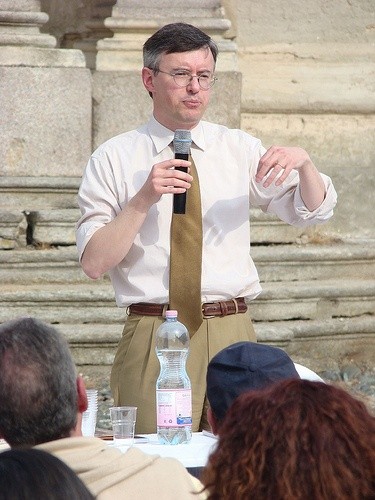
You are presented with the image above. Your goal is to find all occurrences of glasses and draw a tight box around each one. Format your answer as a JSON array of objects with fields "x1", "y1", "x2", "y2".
[{"x1": 151, "y1": 68, "x2": 218, "y2": 89}]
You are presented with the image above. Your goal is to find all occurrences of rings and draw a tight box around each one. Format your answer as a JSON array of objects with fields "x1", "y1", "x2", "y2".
[{"x1": 276, "y1": 163, "x2": 284, "y2": 168}]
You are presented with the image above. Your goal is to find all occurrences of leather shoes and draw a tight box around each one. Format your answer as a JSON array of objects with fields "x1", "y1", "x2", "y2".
[{"x1": 129, "y1": 297, "x2": 248, "y2": 317}]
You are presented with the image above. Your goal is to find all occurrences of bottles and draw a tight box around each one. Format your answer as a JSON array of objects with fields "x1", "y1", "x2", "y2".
[{"x1": 156, "y1": 310, "x2": 192, "y2": 446}]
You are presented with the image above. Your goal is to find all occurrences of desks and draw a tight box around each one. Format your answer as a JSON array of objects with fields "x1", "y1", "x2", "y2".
[{"x1": 102, "y1": 430, "x2": 218, "y2": 468}]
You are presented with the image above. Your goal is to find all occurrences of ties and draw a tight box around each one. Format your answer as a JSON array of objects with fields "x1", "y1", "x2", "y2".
[{"x1": 168, "y1": 146, "x2": 204, "y2": 346}]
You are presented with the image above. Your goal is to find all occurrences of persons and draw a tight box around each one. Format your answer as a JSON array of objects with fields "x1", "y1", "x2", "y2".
[
  {"x1": 0, "y1": 318, "x2": 374, "y2": 500},
  {"x1": 76, "y1": 23, "x2": 338, "y2": 434}
]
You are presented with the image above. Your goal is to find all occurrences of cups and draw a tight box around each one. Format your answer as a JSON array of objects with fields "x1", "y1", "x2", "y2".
[
  {"x1": 108, "y1": 406, "x2": 138, "y2": 444},
  {"x1": 81, "y1": 390, "x2": 98, "y2": 437}
]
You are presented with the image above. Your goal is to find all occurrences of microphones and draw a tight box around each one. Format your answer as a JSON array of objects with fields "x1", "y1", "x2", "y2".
[{"x1": 172, "y1": 129, "x2": 191, "y2": 214}]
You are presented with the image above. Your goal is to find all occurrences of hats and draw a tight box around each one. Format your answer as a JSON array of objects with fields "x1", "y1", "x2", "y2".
[{"x1": 206, "y1": 341, "x2": 301, "y2": 427}]
